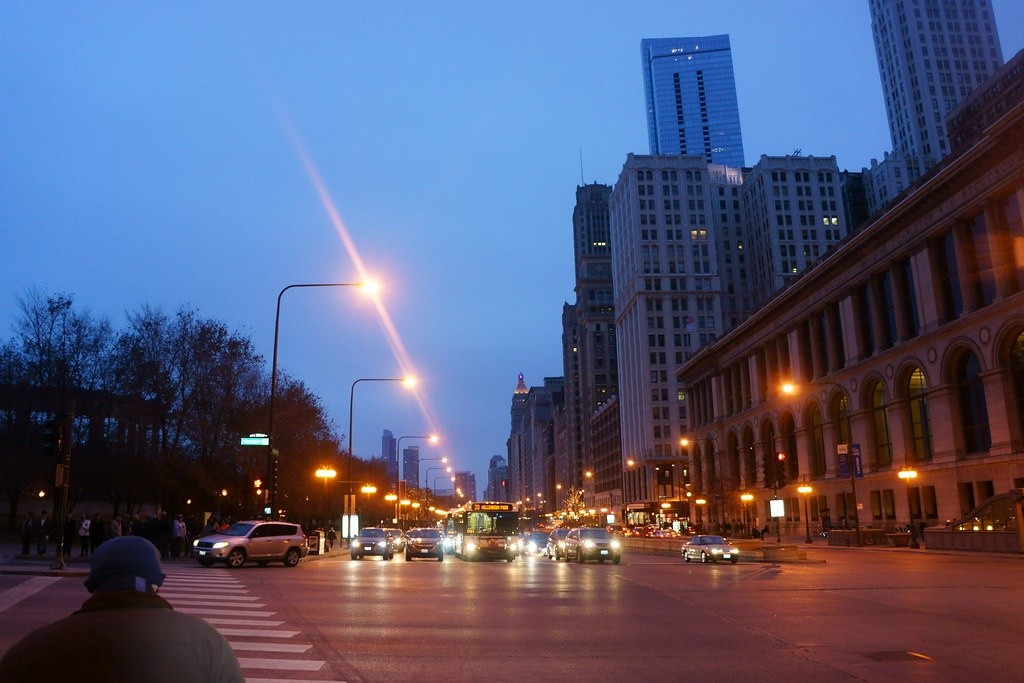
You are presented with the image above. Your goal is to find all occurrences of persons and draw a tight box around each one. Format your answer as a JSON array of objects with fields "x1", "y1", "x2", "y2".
[
  {"x1": 734, "y1": 522, "x2": 744, "y2": 534},
  {"x1": 0, "y1": 535, "x2": 245, "y2": 683},
  {"x1": 110, "y1": 513, "x2": 197, "y2": 557},
  {"x1": 204, "y1": 517, "x2": 230, "y2": 535},
  {"x1": 22, "y1": 510, "x2": 50, "y2": 558},
  {"x1": 327, "y1": 527, "x2": 336, "y2": 547},
  {"x1": 722, "y1": 522, "x2": 732, "y2": 533},
  {"x1": 78, "y1": 513, "x2": 104, "y2": 558},
  {"x1": 63, "y1": 511, "x2": 77, "y2": 557},
  {"x1": 919, "y1": 519, "x2": 927, "y2": 541}
]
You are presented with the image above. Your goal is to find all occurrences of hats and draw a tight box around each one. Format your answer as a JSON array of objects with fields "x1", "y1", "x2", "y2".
[{"x1": 84, "y1": 535, "x2": 166, "y2": 592}]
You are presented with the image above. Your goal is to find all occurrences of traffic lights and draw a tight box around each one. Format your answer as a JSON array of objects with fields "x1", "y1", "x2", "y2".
[
  {"x1": 773, "y1": 451, "x2": 786, "y2": 487},
  {"x1": 250, "y1": 476, "x2": 262, "y2": 500},
  {"x1": 762, "y1": 453, "x2": 774, "y2": 490}
]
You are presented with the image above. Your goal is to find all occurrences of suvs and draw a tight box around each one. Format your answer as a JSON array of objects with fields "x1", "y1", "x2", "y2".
[{"x1": 192, "y1": 519, "x2": 311, "y2": 568}]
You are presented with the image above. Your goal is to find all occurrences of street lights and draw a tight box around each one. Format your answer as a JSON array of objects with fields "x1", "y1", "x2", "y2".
[
  {"x1": 444, "y1": 487, "x2": 461, "y2": 508},
  {"x1": 625, "y1": 459, "x2": 668, "y2": 496},
  {"x1": 316, "y1": 470, "x2": 335, "y2": 522},
  {"x1": 417, "y1": 457, "x2": 449, "y2": 525},
  {"x1": 782, "y1": 381, "x2": 861, "y2": 546},
  {"x1": 267, "y1": 281, "x2": 383, "y2": 515},
  {"x1": 412, "y1": 503, "x2": 420, "y2": 521},
  {"x1": 677, "y1": 438, "x2": 727, "y2": 536},
  {"x1": 399, "y1": 499, "x2": 411, "y2": 527},
  {"x1": 694, "y1": 497, "x2": 706, "y2": 535},
  {"x1": 343, "y1": 375, "x2": 420, "y2": 546},
  {"x1": 584, "y1": 471, "x2": 613, "y2": 513},
  {"x1": 425, "y1": 466, "x2": 452, "y2": 526},
  {"x1": 385, "y1": 494, "x2": 396, "y2": 525},
  {"x1": 740, "y1": 494, "x2": 754, "y2": 540},
  {"x1": 432, "y1": 476, "x2": 456, "y2": 507},
  {"x1": 361, "y1": 485, "x2": 377, "y2": 524},
  {"x1": 897, "y1": 468, "x2": 920, "y2": 549},
  {"x1": 796, "y1": 473, "x2": 815, "y2": 544},
  {"x1": 394, "y1": 436, "x2": 441, "y2": 527}
]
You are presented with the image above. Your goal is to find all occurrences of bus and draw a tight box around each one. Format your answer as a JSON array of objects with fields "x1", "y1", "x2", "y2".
[{"x1": 440, "y1": 499, "x2": 521, "y2": 562}]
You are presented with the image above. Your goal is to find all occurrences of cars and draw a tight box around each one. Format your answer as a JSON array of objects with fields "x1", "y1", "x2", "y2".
[
  {"x1": 680, "y1": 534, "x2": 740, "y2": 565},
  {"x1": 518, "y1": 524, "x2": 681, "y2": 565},
  {"x1": 349, "y1": 526, "x2": 446, "y2": 563}
]
